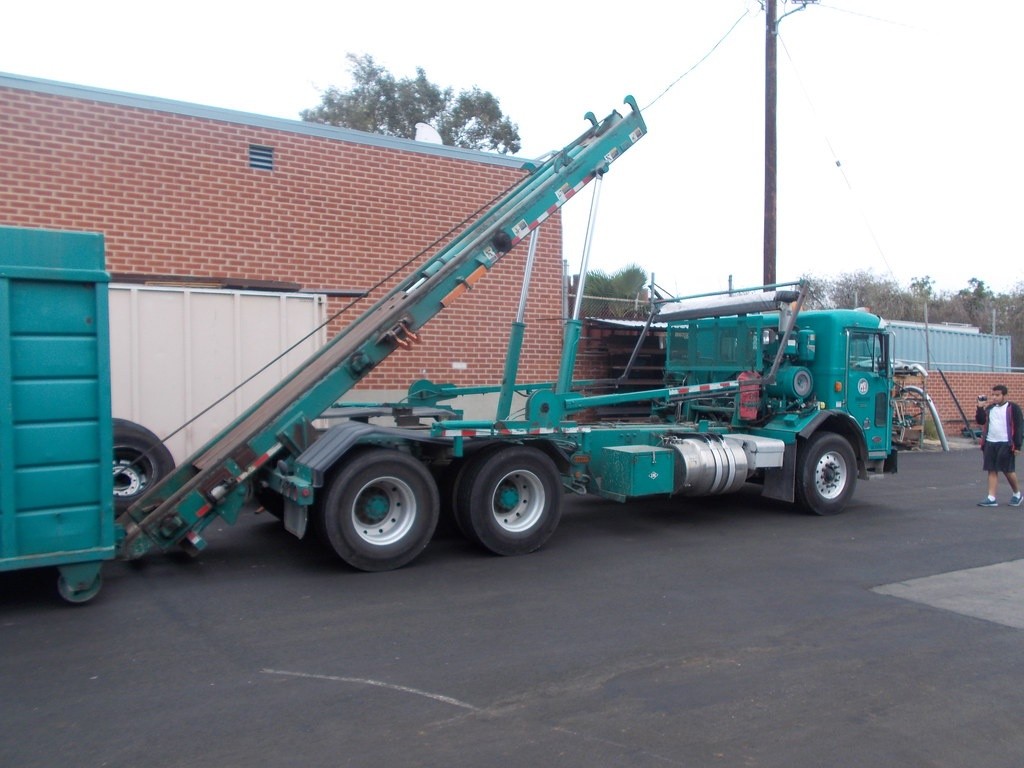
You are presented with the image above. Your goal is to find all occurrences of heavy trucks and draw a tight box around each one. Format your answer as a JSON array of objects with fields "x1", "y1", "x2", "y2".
[{"x1": 116, "y1": 94, "x2": 899, "y2": 573}]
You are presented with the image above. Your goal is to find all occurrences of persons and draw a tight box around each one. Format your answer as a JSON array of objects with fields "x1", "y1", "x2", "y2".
[{"x1": 976, "y1": 386, "x2": 1024, "y2": 507}]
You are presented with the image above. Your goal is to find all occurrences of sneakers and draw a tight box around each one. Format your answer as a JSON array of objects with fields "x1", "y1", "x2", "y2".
[
  {"x1": 976, "y1": 497, "x2": 998, "y2": 506},
  {"x1": 1008, "y1": 492, "x2": 1024, "y2": 507}
]
